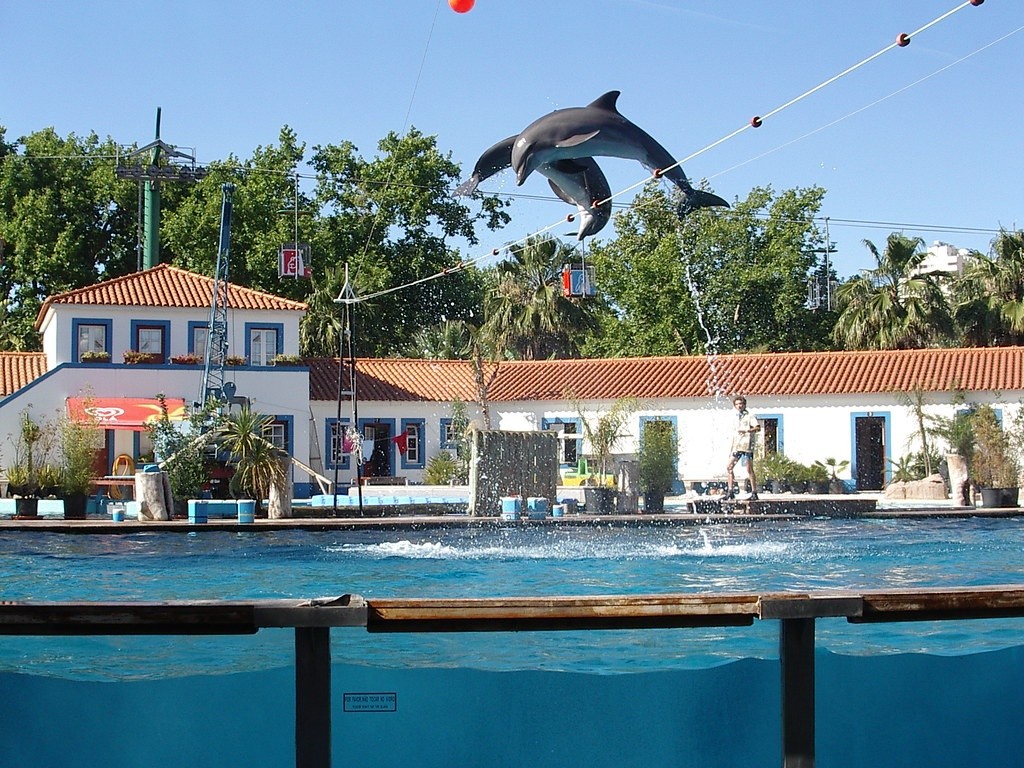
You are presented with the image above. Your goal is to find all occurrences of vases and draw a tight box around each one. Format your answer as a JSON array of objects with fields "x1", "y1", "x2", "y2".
[
  {"x1": 125, "y1": 358, "x2": 138, "y2": 365},
  {"x1": 170, "y1": 358, "x2": 196, "y2": 364},
  {"x1": 82, "y1": 358, "x2": 109, "y2": 363},
  {"x1": 272, "y1": 359, "x2": 296, "y2": 366},
  {"x1": 144, "y1": 358, "x2": 165, "y2": 364}
]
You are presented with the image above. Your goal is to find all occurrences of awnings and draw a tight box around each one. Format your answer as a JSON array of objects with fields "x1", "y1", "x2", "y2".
[{"x1": 67, "y1": 398, "x2": 188, "y2": 431}]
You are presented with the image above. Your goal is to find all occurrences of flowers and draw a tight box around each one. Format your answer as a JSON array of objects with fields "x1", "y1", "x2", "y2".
[
  {"x1": 177, "y1": 352, "x2": 201, "y2": 363},
  {"x1": 274, "y1": 353, "x2": 303, "y2": 363},
  {"x1": 139, "y1": 352, "x2": 154, "y2": 360},
  {"x1": 81, "y1": 351, "x2": 110, "y2": 358},
  {"x1": 121, "y1": 349, "x2": 142, "y2": 363}
]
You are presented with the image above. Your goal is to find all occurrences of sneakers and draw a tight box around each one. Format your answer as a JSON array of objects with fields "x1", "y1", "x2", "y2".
[
  {"x1": 721, "y1": 492, "x2": 735, "y2": 500},
  {"x1": 746, "y1": 494, "x2": 759, "y2": 500}
]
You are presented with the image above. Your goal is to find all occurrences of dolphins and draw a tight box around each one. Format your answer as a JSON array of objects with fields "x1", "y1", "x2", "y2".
[
  {"x1": 511, "y1": 90, "x2": 731, "y2": 222},
  {"x1": 451, "y1": 135, "x2": 612, "y2": 241}
]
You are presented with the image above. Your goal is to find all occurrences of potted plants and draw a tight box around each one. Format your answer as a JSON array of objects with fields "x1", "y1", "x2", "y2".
[
  {"x1": 966, "y1": 402, "x2": 1021, "y2": 508},
  {"x1": 563, "y1": 384, "x2": 681, "y2": 513},
  {"x1": 1, "y1": 389, "x2": 297, "y2": 517},
  {"x1": 752, "y1": 443, "x2": 850, "y2": 493}
]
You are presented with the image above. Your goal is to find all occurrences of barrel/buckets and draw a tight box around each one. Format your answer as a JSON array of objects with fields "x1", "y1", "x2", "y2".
[
  {"x1": 553, "y1": 505, "x2": 563, "y2": 517},
  {"x1": 113, "y1": 509, "x2": 125, "y2": 521}
]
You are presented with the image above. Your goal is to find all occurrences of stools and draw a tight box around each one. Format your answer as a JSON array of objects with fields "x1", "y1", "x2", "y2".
[
  {"x1": 501, "y1": 498, "x2": 522, "y2": 519},
  {"x1": 552, "y1": 505, "x2": 563, "y2": 517},
  {"x1": 188, "y1": 500, "x2": 207, "y2": 524},
  {"x1": 527, "y1": 497, "x2": 547, "y2": 520},
  {"x1": 113, "y1": 508, "x2": 124, "y2": 521},
  {"x1": 237, "y1": 499, "x2": 255, "y2": 523}
]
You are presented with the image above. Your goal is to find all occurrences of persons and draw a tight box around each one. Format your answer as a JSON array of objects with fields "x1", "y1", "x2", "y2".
[{"x1": 723, "y1": 396, "x2": 761, "y2": 501}]
